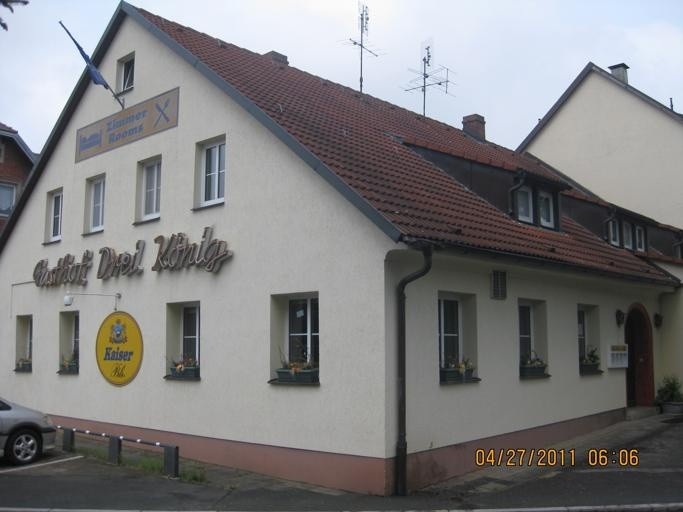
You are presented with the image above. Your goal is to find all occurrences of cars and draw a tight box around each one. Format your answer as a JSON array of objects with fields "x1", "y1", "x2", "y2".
[{"x1": 0, "y1": 395, "x2": 57, "y2": 466}]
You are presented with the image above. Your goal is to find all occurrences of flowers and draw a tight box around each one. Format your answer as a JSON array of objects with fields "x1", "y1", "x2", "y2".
[{"x1": 288, "y1": 362, "x2": 300, "y2": 376}]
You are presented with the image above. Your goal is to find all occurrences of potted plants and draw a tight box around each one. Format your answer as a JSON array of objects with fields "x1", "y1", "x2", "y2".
[
  {"x1": 519, "y1": 350, "x2": 547, "y2": 377},
  {"x1": 650, "y1": 376, "x2": 683, "y2": 414},
  {"x1": 580, "y1": 346, "x2": 601, "y2": 371},
  {"x1": 440, "y1": 356, "x2": 461, "y2": 382},
  {"x1": 59, "y1": 348, "x2": 78, "y2": 374},
  {"x1": 463, "y1": 359, "x2": 476, "y2": 378},
  {"x1": 299, "y1": 361, "x2": 317, "y2": 384}
]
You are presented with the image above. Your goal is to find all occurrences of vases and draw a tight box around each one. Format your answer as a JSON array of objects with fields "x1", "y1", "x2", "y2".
[
  {"x1": 14, "y1": 357, "x2": 31, "y2": 373},
  {"x1": 275, "y1": 368, "x2": 292, "y2": 382},
  {"x1": 170, "y1": 367, "x2": 200, "y2": 380}
]
